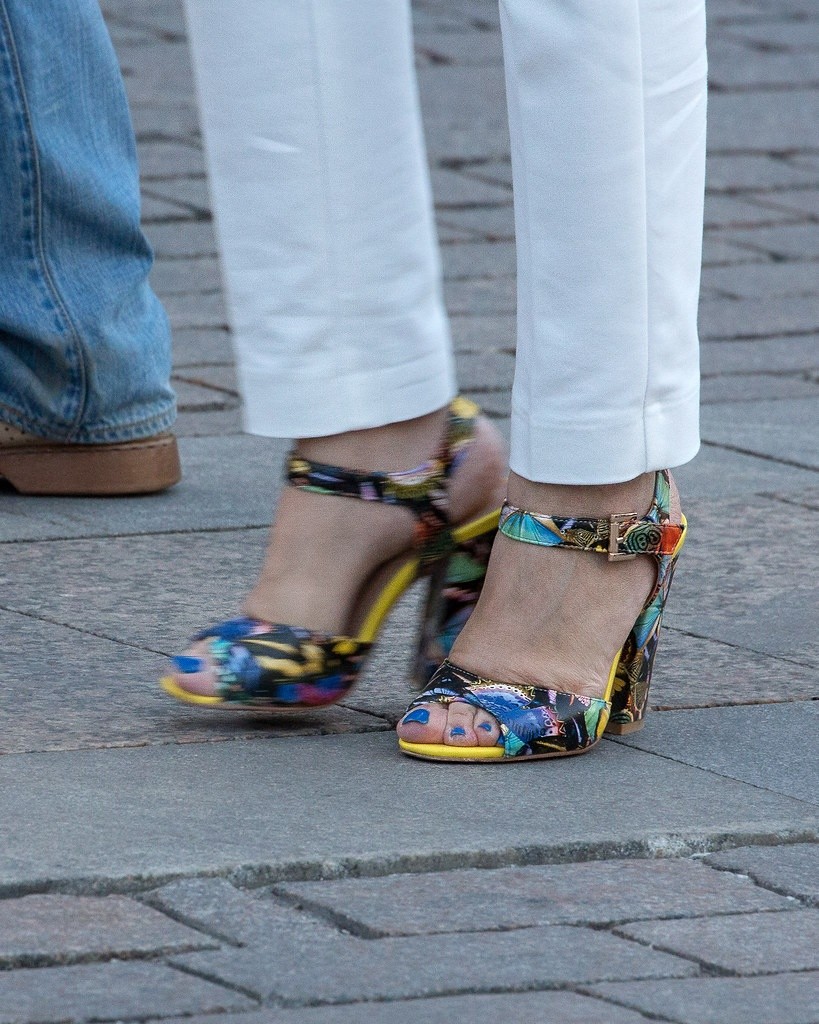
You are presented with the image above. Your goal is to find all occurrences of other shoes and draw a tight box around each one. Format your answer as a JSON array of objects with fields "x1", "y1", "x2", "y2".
[{"x1": 0, "y1": 419, "x2": 181, "y2": 495}]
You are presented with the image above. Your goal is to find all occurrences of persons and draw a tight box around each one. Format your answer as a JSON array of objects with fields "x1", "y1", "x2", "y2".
[
  {"x1": 156, "y1": 0, "x2": 710, "y2": 762},
  {"x1": 2, "y1": 1, "x2": 182, "y2": 498}
]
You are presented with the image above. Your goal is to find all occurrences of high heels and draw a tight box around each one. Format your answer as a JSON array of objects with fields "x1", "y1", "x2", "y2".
[
  {"x1": 396, "y1": 468, "x2": 688, "y2": 762},
  {"x1": 159, "y1": 397, "x2": 502, "y2": 712}
]
